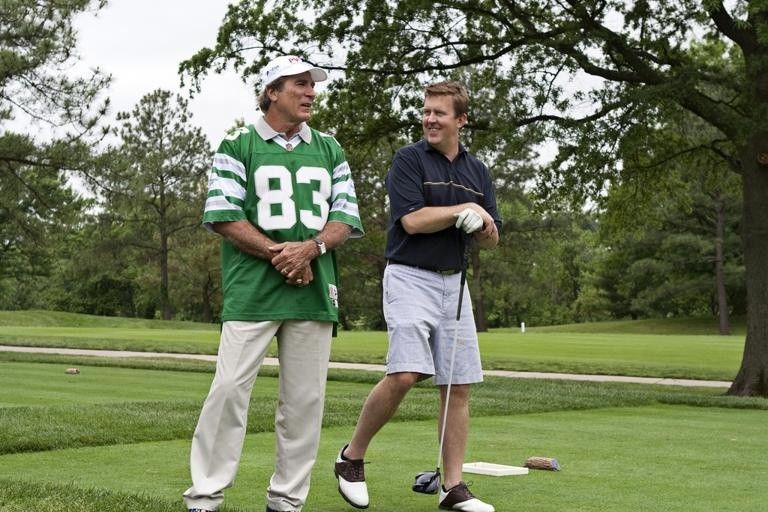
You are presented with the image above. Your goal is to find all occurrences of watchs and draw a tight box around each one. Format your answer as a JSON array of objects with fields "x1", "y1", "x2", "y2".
[{"x1": 312, "y1": 238, "x2": 327, "y2": 256}]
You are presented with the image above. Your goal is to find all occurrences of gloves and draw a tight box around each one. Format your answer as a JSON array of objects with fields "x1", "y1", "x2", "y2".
[{"x1": 453, "y1": 207, "x2": 484, "y2": 234}]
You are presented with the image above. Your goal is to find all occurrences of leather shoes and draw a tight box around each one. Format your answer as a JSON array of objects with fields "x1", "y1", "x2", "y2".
[
  {"x1": 436, "y1": 480, "x2": 497, "y2": 512},
  {"x1": 332, "y1": 444, "x2": 371, "y2": 509}
]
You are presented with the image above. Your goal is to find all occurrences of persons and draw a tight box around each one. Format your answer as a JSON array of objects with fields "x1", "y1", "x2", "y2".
[
  {"x1": 333, "y1": 80, "x2": 503, "y2": 512},
  {"x1": 181, "y1": 55, "x2": 365, "y2": 512}
]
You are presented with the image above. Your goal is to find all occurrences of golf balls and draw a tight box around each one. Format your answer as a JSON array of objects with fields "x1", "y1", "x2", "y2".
[{"x1": 417, "y1": 473, "x2": 438, "y2": 492}]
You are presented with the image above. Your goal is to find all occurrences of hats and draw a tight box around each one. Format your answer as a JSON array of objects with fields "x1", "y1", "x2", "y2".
[{"x1": 259, "y1": 53, "x2": 328, "y2": 87}]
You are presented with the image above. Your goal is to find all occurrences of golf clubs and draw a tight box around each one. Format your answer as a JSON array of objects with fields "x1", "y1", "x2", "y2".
[{"x1": 411, "y1": 245, "x2": 469, "y2": 494}]
